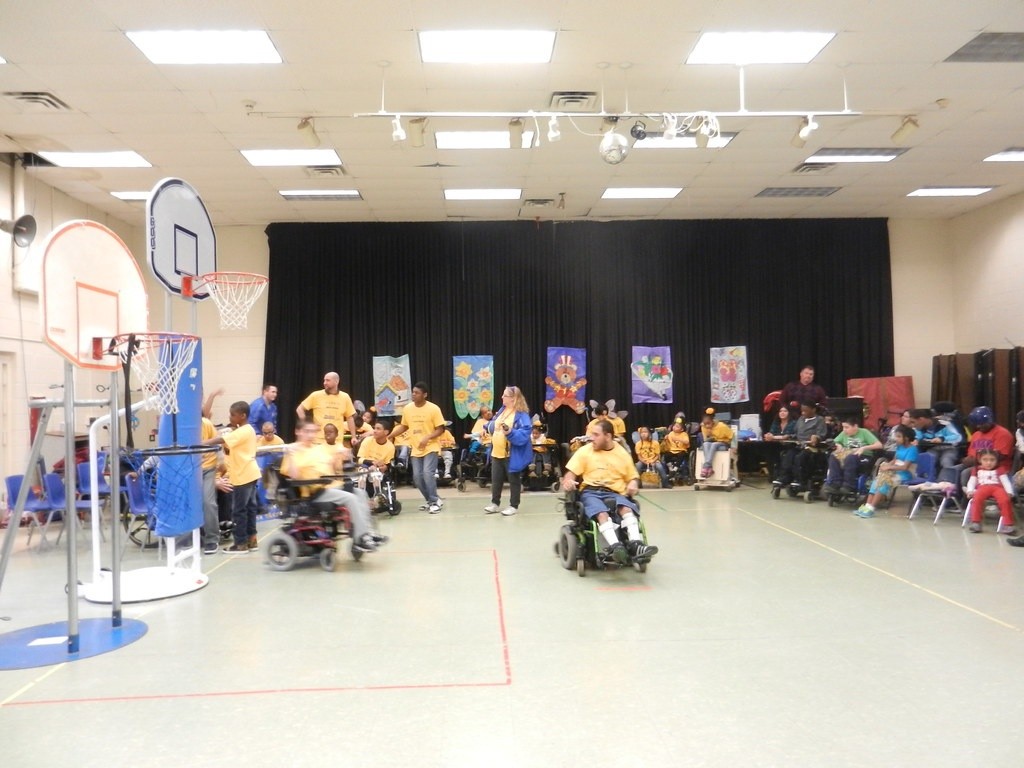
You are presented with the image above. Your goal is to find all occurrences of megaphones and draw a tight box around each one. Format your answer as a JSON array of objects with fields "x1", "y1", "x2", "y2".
[{"x1": 0, "y1": 215, "x2": 36, "y2": 247}]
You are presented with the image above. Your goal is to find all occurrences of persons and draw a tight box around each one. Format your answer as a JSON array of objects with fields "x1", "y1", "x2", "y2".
[
  {"x1": 296, "y1": 372, "x2": 359, "y2": 447},
  {"x1": 563, "y1": 420, "x2": 658, "y2": 565},
  {"x1": 281, "y1": 415, "x2": 389, "y2": 553},
  {"x1": 824, "y1": 416, "x2": 883, "y2": 493},
  {"x1": 585, "y1": 404, "x2": 626, "y2": 438},
  {"x1": 387, "y1": 382, "x2": 445, "y2": 514},
  {"x1": 961, "y1": 406, "x2": 1014, "y2": 509},
  {"x1": 343, "y1": 409, "x2": 456, "y2": 478},
  {"x1": 778, "y1": 364, "x2": 827, "y2": 419},
  {"x1": 480, "y1": 386, "x2": 533, "y2": 515},
  {"x1": 699, "y1": 407, "x2": 733, "y2": 479},
  {"x1": 967, "y1": 449, "x2": 1016, "y2": 534},
  {"x1": 764, "y1": 399, "x2": 828, "y2": 486},
  {"x1": 356, "y1": 420, "x2": 395, "y2": 510},
  {"x1": 318, "y1": 424, "x2": 348, "y2": 481},
  {"x1": 884, "y1": 402, "x2": 971, "y2": 513},
  {"x1": 128, "y1": 385, "x2": 283, "y2": 554},
  {"x1": 853, "y1": 425, "x2": 919, "y2": 518},
  {"x1": 634, "y1": 412, "x2": 690, "y2": 489},
  {"x1": 528, "y1": 420, "x2": 555, "y2": 477},
  {"x1": 1006, "y1": 411, "x2": 1024, "y2": 546},
  {"x1": 466, "y1": 405, "x2": 493, "y2": 465}
]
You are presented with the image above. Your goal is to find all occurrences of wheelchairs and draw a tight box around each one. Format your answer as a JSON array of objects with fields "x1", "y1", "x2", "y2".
[
  {"x1": 457, "y1": 439, "x2": 496, "y2": 492},
  {"x1": 390, "y1": 448, "x2": 457, "y2": 488},
  {"x1": 554, "y1": 477, "x2": 648, "y2": 576},
  {"x1": 661, "y1": 450, "x2": 697, "y2": 486},
  {"x1": 823, "y1": 454, "x2": 880, "y2": 507},
  {"x1": 343, "y1": 458, "x2": 403, "y2": 517},
  {"x1": 262, "y1": 481, "x2": 389, "y2": 571},
  {"x1": 521, "y1": 443, "x2": 560, "y2": 493},
  {"x1": 121, "y1": 488, "x2": 236, "y2": 549},
  {"x1": 771, "y1": 441, "x2": 831, "y2": 502}
]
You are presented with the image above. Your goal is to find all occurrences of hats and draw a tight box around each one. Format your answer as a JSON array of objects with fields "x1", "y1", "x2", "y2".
[{"x1": 930, "y1": 401, "x2": 956, "y2": 416}]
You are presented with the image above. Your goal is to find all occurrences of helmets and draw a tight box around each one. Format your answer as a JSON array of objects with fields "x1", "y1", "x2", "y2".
[{"x1": 968, "y1": 405, "x2": 993, "y2": 424}]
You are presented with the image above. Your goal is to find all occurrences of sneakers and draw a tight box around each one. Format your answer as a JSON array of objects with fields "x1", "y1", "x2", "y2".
[
  {"x1": 204, "y1": 543, "x2": 219, "y2": 554},
  {"x1": 626, "y1": 540, "x2": 658, "y2": 559},
  {"x1": 420, "y1": 497, "x2": 444, "y2": 514},
  {"x1": 484, "y1": 503, "x2": 500, "y2": 513},
  {"x1": 609, "y1": 541, "x2": 629, "y2": 561},
  {"x1": 501, "y1": 506, "x2": 518, "y2": 516},
  {"x1": 853, "y1": 504, "x2": 874, "y2": 518},
  {"x1": 247, "y1": 535, "x2": 257, "y2": 550},
  {"x1": 701, "y1": 467, "x2": 713, "y2": 478},
  {"x1": 353, "y1": 533, "x2": 388, "y2": 554},
  {"x1": 222, "y1": 542, "x2": 249, "y2": 555}
]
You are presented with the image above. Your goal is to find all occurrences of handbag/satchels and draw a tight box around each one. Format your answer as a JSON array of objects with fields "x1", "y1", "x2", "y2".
[{"x1": 641, "y1": 462, "x2": 662, "y2": 488}]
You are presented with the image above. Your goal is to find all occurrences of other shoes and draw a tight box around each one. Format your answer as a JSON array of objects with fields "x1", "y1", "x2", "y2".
[
  {"x1": 932, "y1": 502, "x2": 961, "y2": 514},
  {"x1": 839, "y1": 486, "x2": 858, "y2": 496},
  {"x1": 443, "y1": 470, "x2": 451, "y2": 478},
  {"x1": 528, "y1": 470, "x2": 536, "y2": 477},
  {"x1": 790, "y1": 478, "x2": 801, "y2": 487},
  {"x1": 1002, "y1": 524, "x2": 1016, "y2": 534},
  {"x1": 1007, "y1": 535, "x2": 1024, "y2": 547},
  {"x1": 772, "y1": 477, "x2": 784, "y2": 486},
  {"x1": 969, "y1": 522, "x2": 982, "y2": 533},
  {"x1": 218, "y1": 520, "x2": 237, "y2": 533},
  {"x1": 824, "y1": 485, "x2": 840, "y2": 494},
  {"x1": 434, "y1": 469, "x2": 439, "y2": 479},
  {"x1": 543, "y1": 469, "x2": 551, "y2": 477}
]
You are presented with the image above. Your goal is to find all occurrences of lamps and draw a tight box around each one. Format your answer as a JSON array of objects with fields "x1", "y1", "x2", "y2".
[
  {"x1": 409, "y1": 116, "x2": 430, "y2": 148},
  {"x1": 890, "y1": 115, "x2": 919, "y2": 146},
  {"x1": 630, "y1": 120, "x2": 647, "y2": 140},
  {"x1": 597, "y1": 117, "x2": 619, "y2": 144},
  {"x1": 548, "y1": 116, "x2": 560, "y2": 142},
  {"x1": 297, "y1": 116, "x2": 321, "y2": 148},
  {"x1": 509, "y1": 116, "x2": 527, "y2": 150},
  {"x1": 799, "y1": 115, "x2": 819, "y2": 140},
  {"x1": 392, "y1": 114, "x2": 407, "y2": 141},
  {"x1": 790, "y1": 115, "x2": 808, "y2": 148},
  {"x1": 556, "y1": 192, "x2": 566, "y2": 209}
]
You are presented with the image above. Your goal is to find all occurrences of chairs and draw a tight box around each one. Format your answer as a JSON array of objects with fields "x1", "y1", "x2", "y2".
[
  {"x1": 881, "y1": 441, "x2": 1022, "y2": 532},
  {"x1": 695, "y1": 425, "x2": 738, "y2": 482},
  {"x1": 4, "y1": 450, "x2": 163, "y2": 566}
]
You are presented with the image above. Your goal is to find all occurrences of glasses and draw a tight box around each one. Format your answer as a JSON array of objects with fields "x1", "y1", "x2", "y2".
[
  {"x1": 263, "y1": 430, "x2": 273, "y2": 435},
  {"x1": 503, "y1": 392, "x2": 513, "y2": 397}
]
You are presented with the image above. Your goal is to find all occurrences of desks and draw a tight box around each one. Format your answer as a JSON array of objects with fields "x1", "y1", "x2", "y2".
[
  {"x1": 738, "y1": 440, "x2": 780, "y2": 471},
  {"x1": 41, "y1": 430, "x2": 89, "y2": 472}
]
